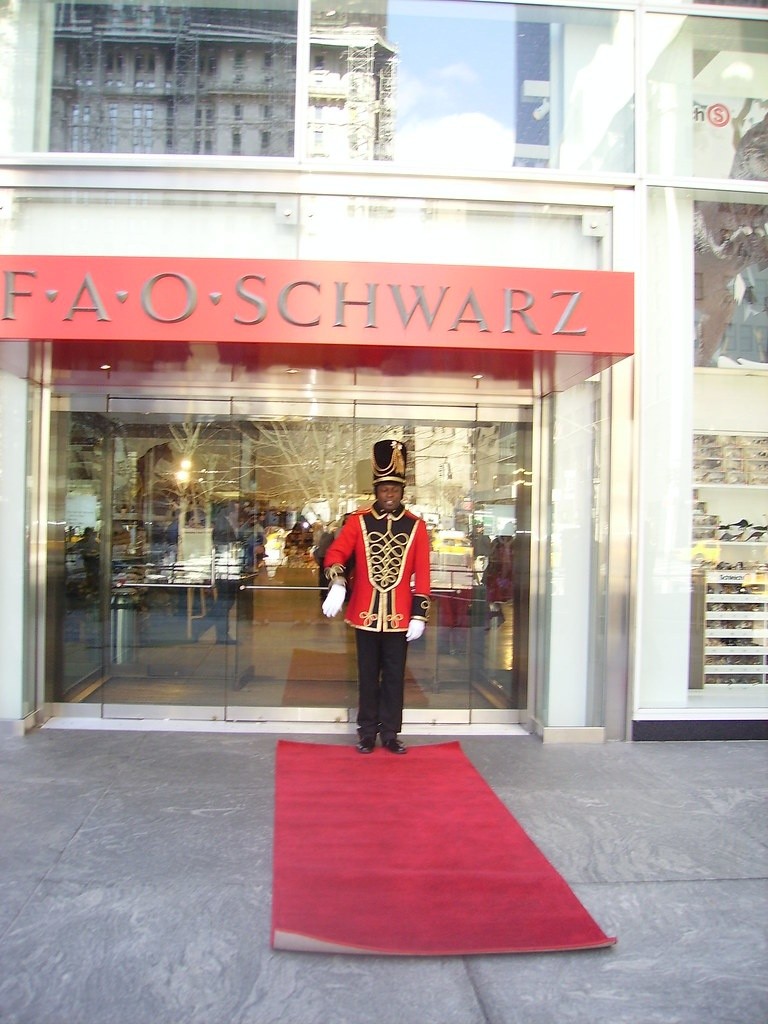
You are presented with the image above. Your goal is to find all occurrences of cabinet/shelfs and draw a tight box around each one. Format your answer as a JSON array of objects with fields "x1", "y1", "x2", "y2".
[{"x1": 688, "y1": 568, "x2": 768, "y2": 690}]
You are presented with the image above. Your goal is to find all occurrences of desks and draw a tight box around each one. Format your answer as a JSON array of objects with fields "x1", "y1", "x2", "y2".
[{"x1": 255, "y1": 564, "x2": 357, "y2": 683}]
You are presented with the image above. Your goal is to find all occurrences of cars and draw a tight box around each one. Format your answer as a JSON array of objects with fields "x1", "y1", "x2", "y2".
[{"x1": 409, "y1": 501, "x2": 515, "y2": 601}]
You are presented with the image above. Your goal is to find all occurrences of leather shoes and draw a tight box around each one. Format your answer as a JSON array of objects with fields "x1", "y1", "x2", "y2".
[
  {"x1": 382, "y1": 739, "x2": 407, "y2": 754},
  {"x1": 355, "y1": 737, "x2": 376, "y2": 752}
]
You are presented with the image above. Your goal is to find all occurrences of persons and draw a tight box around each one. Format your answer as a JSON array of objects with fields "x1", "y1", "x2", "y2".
[
  {"x1": 477, "y1": 523, "x2": 512, "y2": 632},
  {"x1": 322, "y1": 440, "x2": 432, "y2": 755},
  {"x1": 67, "y1": 501, "x2": 353, "y2": 645}
]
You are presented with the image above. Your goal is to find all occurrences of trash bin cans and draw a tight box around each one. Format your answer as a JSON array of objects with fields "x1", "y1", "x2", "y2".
[{"x1": 111, "y1": 587, "x2": 136, "y2": 665}]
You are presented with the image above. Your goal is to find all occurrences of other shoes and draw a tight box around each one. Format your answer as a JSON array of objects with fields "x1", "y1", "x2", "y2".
[
  {"x1": 216, "y1": 638, "x2": 239, "y2": 645},
  {"x1": 190, "y1": 620, "x2": 200, "y2": 641}
]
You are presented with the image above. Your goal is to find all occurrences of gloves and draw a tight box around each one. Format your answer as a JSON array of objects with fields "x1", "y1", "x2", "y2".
[
  {"x1": 405, "y1": 619, "x2": 425, "y2": 642},
  {"x1": 321, "y1": 584, "x2": 346, "y2": 616}
]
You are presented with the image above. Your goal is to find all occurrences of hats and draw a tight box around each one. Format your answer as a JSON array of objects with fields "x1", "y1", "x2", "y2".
[{"x1": 372, "y1": 440, "x2": 407, "y2": 486}]
